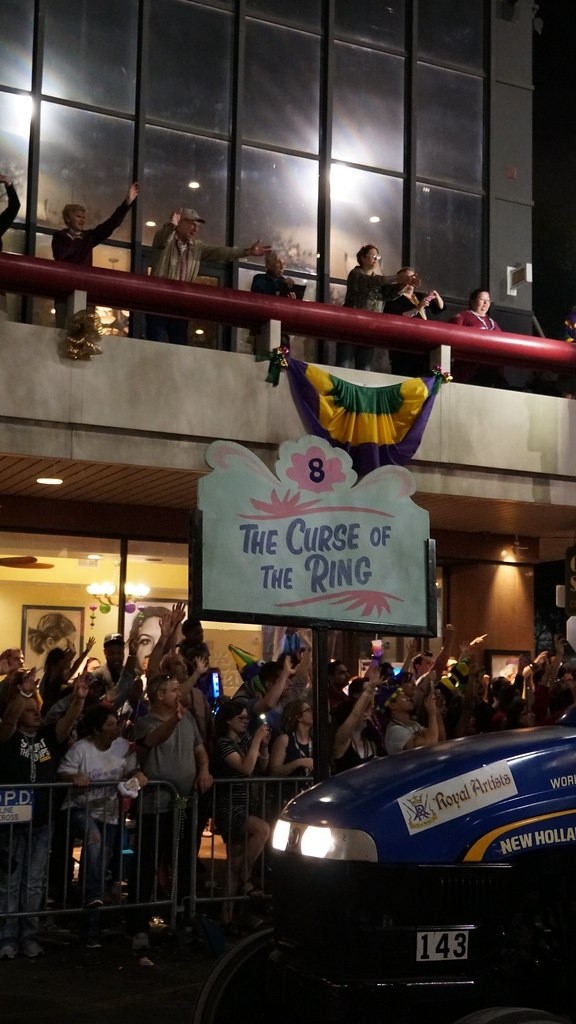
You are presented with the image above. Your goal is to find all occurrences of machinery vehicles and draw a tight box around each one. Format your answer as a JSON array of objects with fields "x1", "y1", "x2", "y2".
[{"x1": 177, "y1": 695, "x2": 576, "y2": 1022}]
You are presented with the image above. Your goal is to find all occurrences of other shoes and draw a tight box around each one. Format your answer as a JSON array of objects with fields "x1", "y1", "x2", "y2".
[
  {"x1": 246, "y1": 915, "x2": 265, "y2": 928},
  {"x1": 238, "y1": 879, "x2": 265, "y2": 897},
  {"x1": 0, "y1": 942, "x2": 19, "y2": 959},
  {"x1": 85, "y1": 896, "x2": 104, "y2": 907},
  {"x1": 20, "y1": 940, "x2": 44, "y2": 958},
  {"x1": 219, "y1": 923, "x2": 248, "y2": 938},
  {"x1": 83, "y1": 936, "x2": 103, "y2": 948},
  {"x1": 132, "y1": 932, "x2": 150, "y2": 950}
]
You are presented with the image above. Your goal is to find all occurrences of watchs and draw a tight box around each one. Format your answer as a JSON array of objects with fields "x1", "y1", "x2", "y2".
[{"x1": 529, "y1": 661, "x2": 539, "y2": 671}]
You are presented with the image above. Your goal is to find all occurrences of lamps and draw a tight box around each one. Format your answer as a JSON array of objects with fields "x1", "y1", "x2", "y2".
[{"x1": 86, "y1": 560, "x2": 150, "y2": 607}]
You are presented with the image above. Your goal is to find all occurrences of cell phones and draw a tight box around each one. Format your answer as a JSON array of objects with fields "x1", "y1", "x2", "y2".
[
  {"x1": 260, "y1": 714, "x2": 267, "y2": 726},
  {"x1": 425, "y1": 295, "x2": 435, "y2": 301},
  {"x1": 431, "y1": 681, "x2": 435, "y2": 696}
]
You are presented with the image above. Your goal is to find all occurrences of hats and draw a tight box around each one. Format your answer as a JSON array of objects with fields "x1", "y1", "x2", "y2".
[
  {"x1": 181, "y1": 209, "x2": 207, "y2": 224},
  {"x1": 104, "y1": 633, "x2": 125, "y2": 647},
  {"x1": 229, "y1": 644, "x2": 266, "y2": 682},
  {"x1": 375, "y1": 686, "x2": 403, "y2": 709}
]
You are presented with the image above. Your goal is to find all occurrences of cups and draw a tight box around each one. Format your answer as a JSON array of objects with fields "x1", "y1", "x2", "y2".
[{"x1": 371, "y1": 639, "x2": 382, "y2": 657}]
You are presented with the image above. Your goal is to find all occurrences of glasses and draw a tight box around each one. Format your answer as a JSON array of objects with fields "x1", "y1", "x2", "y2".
[
  {"x1": 300, "y1": 708, "x2": 312, "y2": 714},
  {"x1": 155, "y1": 673, "x2": 174, "y2": 693},
  {"x1": 364, "y1": 255, "x2": 381, "y2": 260}
]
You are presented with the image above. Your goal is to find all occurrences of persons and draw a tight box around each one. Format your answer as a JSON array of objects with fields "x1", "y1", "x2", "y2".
[
  {"x1": 0, "y1": 599, "x2": 576, "y2": 965},
  {"x1": 452, "y1": 289, "x2": 518, "y2": 392},
  {"x1": 250, "y1": 249, "x2": 298, "y2": 355},
  {"x1": 51, "y1": 180, "x2": 140, "y2": 329},
  {"x1": 0, "y1": 173, "x2": 22, "y2": 251},
  {"x1": 143, "y1": 207, "x2": 272, "y2": 346},
  {"x1": 382, "y1": 267, "x2": 446, "y2": 379},
  {"x1": 563, "y1": 305, "x2": 576, "y2": 400},
  {"x1": 25, "y1": 611, "x2": 77, "y2": 685},
  {"x1": 123, "y1": 605, "x2": 185, "y2": 693},
  {"x1": 334, "y1": 244, "x2": 422, "y2": 371}
]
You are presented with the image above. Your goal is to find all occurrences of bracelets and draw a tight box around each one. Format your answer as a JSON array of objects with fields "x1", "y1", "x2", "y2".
[
  {"x1": 366, "y1": 687, "x2": 375, "y2": 693},
  {"x1": 19, "y1": 689, "x2": 32, "y2": 698},
  {"x1": 371, "y1": 654, "x2": 379, "y2": 660},
  {"x1": 362, "y1": 692, "x2": 372, "y2": 697}
]
